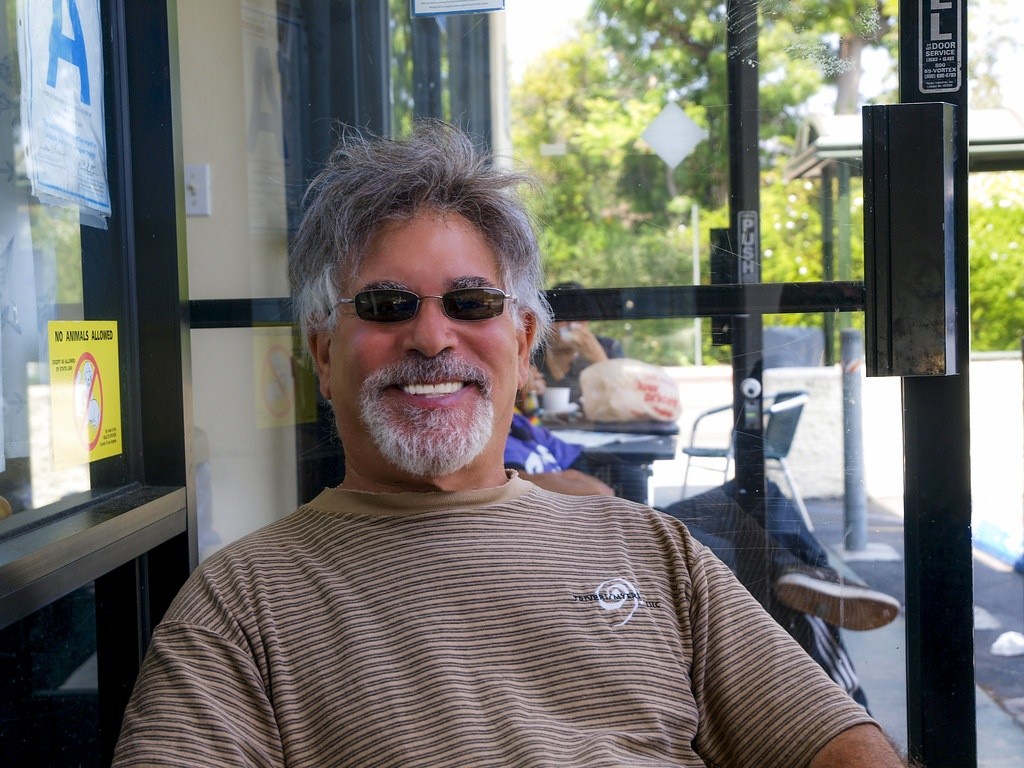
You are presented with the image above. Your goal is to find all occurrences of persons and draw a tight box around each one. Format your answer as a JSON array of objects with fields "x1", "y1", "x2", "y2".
[
  {"x1": 502, "y1": 398, "x2": 903, "y2": 716},
  {"x1": 110, "y1": 116, "x2": 925, "y2": 768},
  {"x1": 529, "y1": 279, "x2": 627, "y2": 425}
]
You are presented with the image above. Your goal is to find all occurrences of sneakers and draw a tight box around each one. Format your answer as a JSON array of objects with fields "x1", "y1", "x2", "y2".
[{"x1": 773, "y1": 565, "x2": 900, "y2": 632}]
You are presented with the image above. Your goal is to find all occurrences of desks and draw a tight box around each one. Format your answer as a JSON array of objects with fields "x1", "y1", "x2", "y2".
[{"x1": 548, "y1": 431, "x2": 676, "y2": 507}]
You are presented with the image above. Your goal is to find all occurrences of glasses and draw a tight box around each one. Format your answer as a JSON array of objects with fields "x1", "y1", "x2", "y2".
[{"x1": 328, "y1": 287, "x2": 520, "y2": 325}]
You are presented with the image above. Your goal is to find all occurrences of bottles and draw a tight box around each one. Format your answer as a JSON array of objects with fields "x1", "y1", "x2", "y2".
[{"x1": 523, "y1": 391, "x2": 540, "y2": 427}]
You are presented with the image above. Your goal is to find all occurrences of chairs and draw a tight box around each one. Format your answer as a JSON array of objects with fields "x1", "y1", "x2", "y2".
[{"x1": 682, "y1": 391, "x2": 815, "y2": 536}]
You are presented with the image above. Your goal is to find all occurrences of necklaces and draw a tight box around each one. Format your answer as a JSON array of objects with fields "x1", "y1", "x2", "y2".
[{"x1": 548, "y1": 348, "x2": 581, "y2": 378}]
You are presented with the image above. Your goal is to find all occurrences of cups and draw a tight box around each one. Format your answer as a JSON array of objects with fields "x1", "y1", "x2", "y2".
[
  {"x1": 543, "y1": 387, "x2": 569, "y2": 411},
  {"x1": 559, "y1": 325, "x2": 583, "y2": 345}
]
